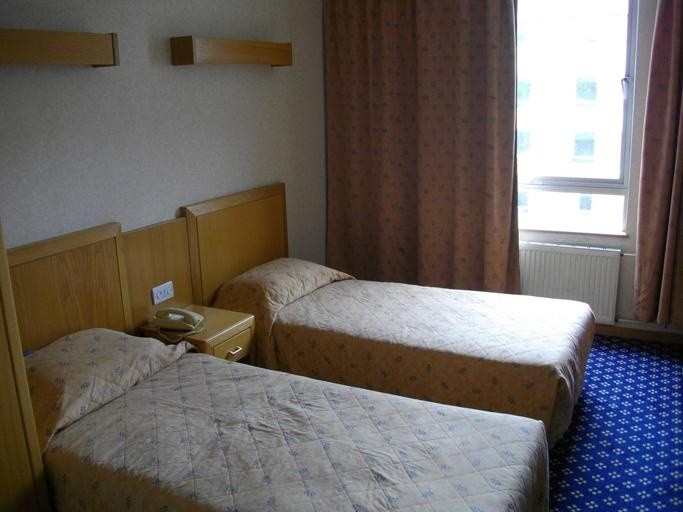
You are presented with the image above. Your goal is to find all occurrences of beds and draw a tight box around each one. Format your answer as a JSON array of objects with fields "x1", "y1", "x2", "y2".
[
  {"x1": 42, "y1": 353, "x2": 551, "y2": 511},
  {"x1": 271, "y1": 277, "x2": 597, "y2": 455}
]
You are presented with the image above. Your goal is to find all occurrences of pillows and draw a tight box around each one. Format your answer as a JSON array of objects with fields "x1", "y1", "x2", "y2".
[
  {"x1": 213, "y1": 257, "x2": 351, "y2": 319},
  {"x1": 23, "y1": 326, "x2": 195, "y2": 455}
]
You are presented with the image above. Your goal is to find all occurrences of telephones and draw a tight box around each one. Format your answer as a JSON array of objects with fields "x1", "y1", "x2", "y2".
[{"x1": 149, "y1": 307, "x2": 204, "y2": 332}]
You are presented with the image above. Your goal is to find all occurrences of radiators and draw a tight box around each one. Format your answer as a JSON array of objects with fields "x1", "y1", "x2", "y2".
[{"x1": 519, "y1": 241, "x2": 624, "y2": 328}]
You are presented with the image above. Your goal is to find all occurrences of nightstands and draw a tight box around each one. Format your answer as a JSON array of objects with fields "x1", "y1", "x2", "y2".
[{"x1": 141, "y1": 303, "x2": 259, "y2": 366}]
